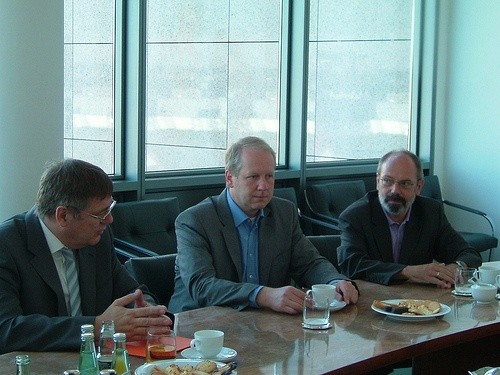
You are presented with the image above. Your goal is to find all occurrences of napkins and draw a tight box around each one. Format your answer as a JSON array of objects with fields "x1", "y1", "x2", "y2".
[{"x1": 102, "y1": 332, "x2": 193, "y2": 357}]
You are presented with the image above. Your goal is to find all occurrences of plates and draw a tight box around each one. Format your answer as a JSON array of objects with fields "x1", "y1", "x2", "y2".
[
  {"x1": 134, "y1": 358, "x2": 238, "y2": 375},
  {"x1": 371, "y1": 298, "x2": 451, "y2": 321},
  {"x1": 180, "y1": 347, "x2": 237, "y2": 362},
  {"x1": 329, "y1": 300, "x2": 349, "y2": 312},
  {"x1": 373, "y1": 315, "x2": 450, "y2": 334}
]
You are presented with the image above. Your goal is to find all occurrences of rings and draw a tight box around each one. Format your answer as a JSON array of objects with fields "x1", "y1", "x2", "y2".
[{"x1": 436, "y1": 271, "x2": 440, "y2": 277}]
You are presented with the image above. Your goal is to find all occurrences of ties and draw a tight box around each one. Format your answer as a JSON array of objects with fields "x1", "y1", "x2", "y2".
[{"x1": 59, "y1": 248, "x2": 83, "y2": 316}]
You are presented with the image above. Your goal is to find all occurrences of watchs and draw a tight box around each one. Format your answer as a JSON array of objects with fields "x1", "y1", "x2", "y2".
[{"x1": 452, "y1": 261, "x2": 468, "y2": 268}]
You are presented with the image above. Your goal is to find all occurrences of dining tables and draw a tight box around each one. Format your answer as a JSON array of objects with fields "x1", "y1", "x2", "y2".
[{"x1": 0, "y1": 280, "x2": 500, "y2": 375}]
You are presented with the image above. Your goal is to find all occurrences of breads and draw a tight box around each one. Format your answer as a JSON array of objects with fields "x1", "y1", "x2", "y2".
[
  {"x1": 148, "y1": 360, "x2": 236, "y2": 375},
  {"x1": 371, "y1": 299, "x2": 441, "y2": 315}
]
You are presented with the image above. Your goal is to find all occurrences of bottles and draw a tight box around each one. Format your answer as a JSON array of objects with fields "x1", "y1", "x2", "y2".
[
  {"x1": 15, "y1": 354, "x2": 31, "y2": 375},
  {"x1": 64, "y1": 319, "x2": 132, "y2": 375}
]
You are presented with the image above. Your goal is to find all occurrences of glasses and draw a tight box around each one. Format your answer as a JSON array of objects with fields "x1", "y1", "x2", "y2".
[
  {"x1": 379, "y1": 177, "x2": 418, "y2": 189},
  {"x1": 66, "y1": 199, "x2": 116, "y2": 220}
]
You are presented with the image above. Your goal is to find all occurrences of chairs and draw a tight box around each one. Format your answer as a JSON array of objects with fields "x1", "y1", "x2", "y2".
[{"x1": 109, "y1": 175, "x2": 498, "y2": 309}]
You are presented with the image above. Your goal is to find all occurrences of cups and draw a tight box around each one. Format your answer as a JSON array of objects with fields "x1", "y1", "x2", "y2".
[
  {"x1": 471, "y1": 284, "x2": 498, "y2": 305},
  {"x1": 190, "y1": 329, "x2": 224, "y2": 357},
  {"x1": 306, "y1": 284, "x2": 336, "y2": 305},
  {"x1": 474, "y1": 267, "x2": 498, "y2": 284},
  {"x1": 303, "y1": 331, "x2": 328, "y2": 360},
  {"x1": 455, "y1": 267, "x2": 477, "y2": 294},
  {"x1": 302, "y1": 289, "x2": 332, "y2": 326},
  {"x1": 470, "y1": 305, "x2": 496, "y2": 321},
  {"x1": 146, "y1": 329, "x2": 176, "y2": 363}
]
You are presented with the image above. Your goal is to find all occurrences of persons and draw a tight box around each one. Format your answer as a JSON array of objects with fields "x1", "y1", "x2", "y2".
[
  {"x1": 170, "y1": 137, "x2": 359, "y2": 314},
  {"x1": 338, "y1": 149, "x2": 480, "y2": 289},
  {"x1": 0, "y1": 158, "x2": 176, "y2": 353}
]
